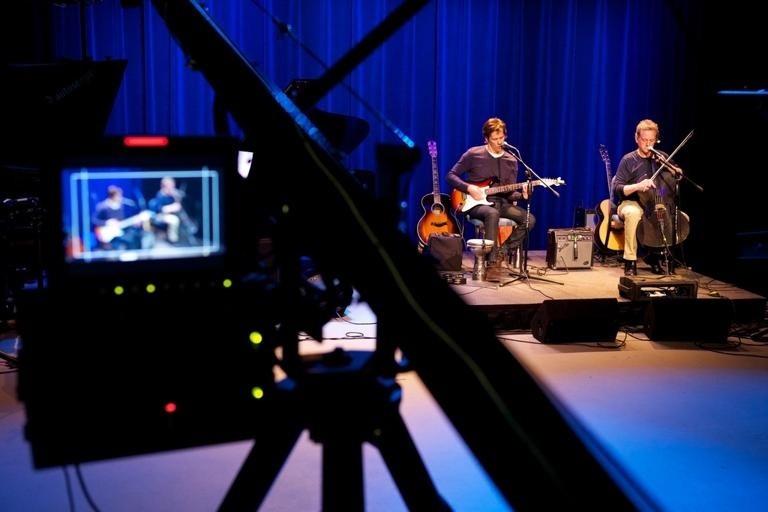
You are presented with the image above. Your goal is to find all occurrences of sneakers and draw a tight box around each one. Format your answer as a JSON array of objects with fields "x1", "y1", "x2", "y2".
[{"x1": 485, "y1": 247, "x2": 507, "y2": 283}]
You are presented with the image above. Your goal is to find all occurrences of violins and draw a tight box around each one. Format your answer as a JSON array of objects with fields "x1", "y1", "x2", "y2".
[{"x1": 636, "y1": 140, "x2": 690, "y2": 248}]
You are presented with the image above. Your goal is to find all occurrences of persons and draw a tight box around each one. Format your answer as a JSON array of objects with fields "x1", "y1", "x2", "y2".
[
  {"x1": 147, "y1": 177, "x2": 185, "y2": 243},
  {"x1": 89, "y1": 185, "x2": 145, "y2": 249},
  {"x1": 446, "y1": 117, "x2": 538, "y2": 283},
  {"x1": 613, "y1": 119, "x2": 683, "y2": 277}
]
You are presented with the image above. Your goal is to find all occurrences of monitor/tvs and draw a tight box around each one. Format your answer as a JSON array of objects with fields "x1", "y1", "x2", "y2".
[{"x1": 58, "y1": 163, "x2": 226, "y2": 267}]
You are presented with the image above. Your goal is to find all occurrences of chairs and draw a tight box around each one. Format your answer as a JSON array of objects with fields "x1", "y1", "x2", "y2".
[
  {"x1": 465, "y1": 210, "x2": 521, "y2": 269},
  {"x1": 602, "y1": 173, "x2": 674, "y2": 277}
]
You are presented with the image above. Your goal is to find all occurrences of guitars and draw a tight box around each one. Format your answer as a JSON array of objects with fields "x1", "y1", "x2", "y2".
[
  {"x1": 95, "y1": 210, "x2": 150, "y2": 244},
  {"x1": 451, "y1": 177, "x2": 565, "y2": 213},
  {"x1": 417, "y1": 142, "x2": 462, "y2": 246},
  {"x1": 594, "y1": 144, "x2": 625, "y2": 255}
]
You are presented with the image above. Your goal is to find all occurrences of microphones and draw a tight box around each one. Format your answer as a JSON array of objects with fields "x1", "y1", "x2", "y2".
[
  {"x1": 647, "y1": 146, "x2": 660, "y2": 155},
  {"x1": 501, "y1": 142, "x2": 516, "y2": 150},
  {"x1": 574, "y1": 237, "x2": 578, "y2": 259}
]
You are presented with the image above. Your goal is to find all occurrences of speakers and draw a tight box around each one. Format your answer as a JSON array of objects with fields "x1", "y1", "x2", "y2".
[
  {"x1": 531, "y1": 297, "x2": 619, "y2": 344},
  {"x1": 428, "y1": 232, "x2": 463, "y2": 271},
  {"x1": 547, "y1": 226, "x2": 594, "y2": 270},
  {"x1": 642, "y1": 296, "x2": 732, "y2": 344}
]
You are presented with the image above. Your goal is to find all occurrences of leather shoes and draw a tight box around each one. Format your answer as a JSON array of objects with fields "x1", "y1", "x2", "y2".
[
  {"x1": 643, "y1": 255, "x2": 666, "y2": 275},
  {"x1": 624, "y1": 260, "x2": 638, "y2": 275}
]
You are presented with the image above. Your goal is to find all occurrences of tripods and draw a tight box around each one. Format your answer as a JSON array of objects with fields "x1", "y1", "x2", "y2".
[
  {"x1": 499, "y1": 149, "x2": 566, "y2": 286},
  {"x1": 213, "y1": 343, "x2": 452, "y2": 512}
]
